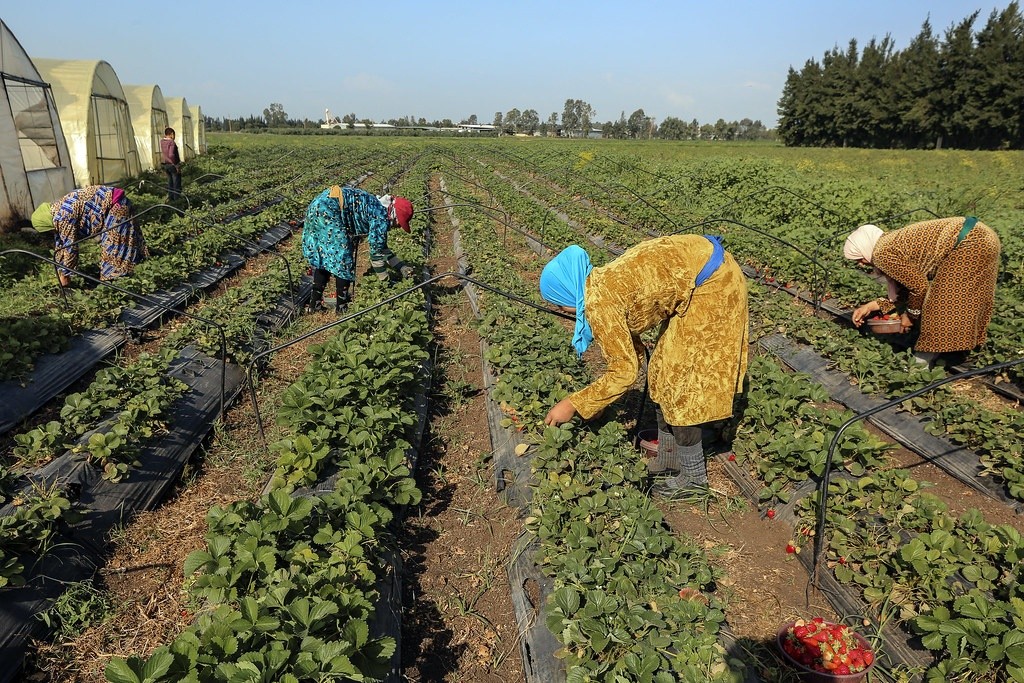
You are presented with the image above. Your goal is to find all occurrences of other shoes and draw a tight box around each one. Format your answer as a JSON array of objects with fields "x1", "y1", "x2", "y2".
[
  {"x1": 308, "y1": 303, "x2": 328, "y2": 315},
  {"x1": 642, "y1": 466, "x2": 681, "y2": 477},
  {"x1": 912, "y1": 353, "x2": 934, "y2": 365},
  {"x1": 335, "y1": 304, "x2": 351, "y2": 315},
  {"x1": 654, "y1": 483, "x2": 708, "y2": 498}
]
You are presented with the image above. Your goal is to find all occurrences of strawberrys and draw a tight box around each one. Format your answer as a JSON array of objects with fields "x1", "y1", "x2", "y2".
[
  {"x1": 783, "y1": 617, "x2": 873, "y2": 676},
  {"x1": 512, "y1": 415, "x2": 517, "y2": 421},
  {"x1": 730, "y1": 454, "x2": 846, "y2": 565},
  {"x1": 20, "y1": 363, "x2": 34, "y2": 388},
  {"x1": 754, "y1": 267, "x2": 901, "y2": 321}
]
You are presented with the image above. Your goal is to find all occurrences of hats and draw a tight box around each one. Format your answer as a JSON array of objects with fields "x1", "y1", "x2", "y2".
[{"x1": 393, "y1": 196, "x2": 414, "y2": 234}]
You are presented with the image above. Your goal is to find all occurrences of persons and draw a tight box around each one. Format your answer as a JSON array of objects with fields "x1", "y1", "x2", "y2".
[
  {"x1": 539, "y1": 234, "x2": 748, "y2": 500},
  {"x1": 31, "y1": 185, "x2": 149, "y2": 286},
  {"x1": 160, "y1": 128, "x2": 182, "y2": 200},
  {"x1": 301, "y1": 185, "x2": 414, "y2": 312},
  {"x1": 842, "y1": 216, "x2": 1001, "y2": 372}
]
